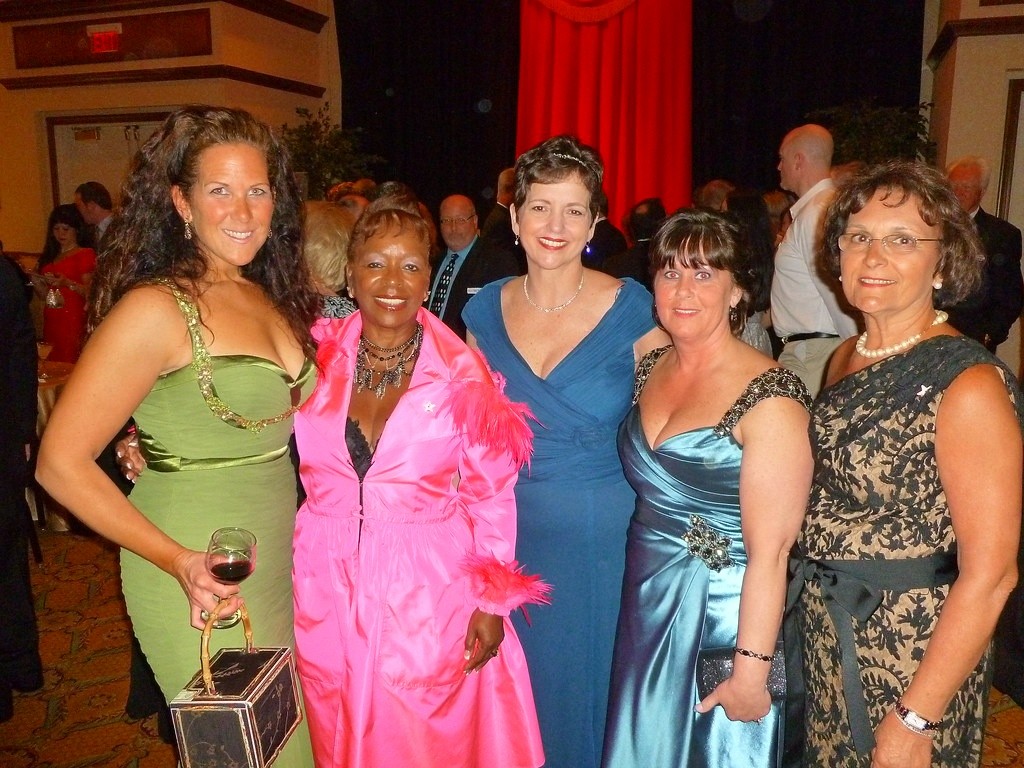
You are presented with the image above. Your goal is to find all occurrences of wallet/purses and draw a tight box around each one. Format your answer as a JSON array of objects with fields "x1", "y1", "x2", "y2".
[{"x1": 698, "y1": 640, "x2": 788, "y2": 703}]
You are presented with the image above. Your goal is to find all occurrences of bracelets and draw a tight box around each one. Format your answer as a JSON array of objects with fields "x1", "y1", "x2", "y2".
[
  {"x1": 894, "y1": 705, "x2": 937, "y2": 737},
  {"x1": 733, "y1": 648, "x2": 775, "y2": 661}
]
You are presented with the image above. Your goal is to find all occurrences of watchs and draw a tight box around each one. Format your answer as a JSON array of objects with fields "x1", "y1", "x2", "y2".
[{"x1": 896, "y1": 697, "x2": 943, "y2": 730}]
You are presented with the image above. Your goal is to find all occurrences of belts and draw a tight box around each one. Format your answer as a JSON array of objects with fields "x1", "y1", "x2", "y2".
[{"x1": 780, "y1": 333, "x2": 841, "y2": 343}]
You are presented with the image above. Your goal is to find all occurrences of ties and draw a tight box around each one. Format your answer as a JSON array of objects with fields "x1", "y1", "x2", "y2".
[
  {"x1": 428, "y1": 254, "x2": 460, "y2": 317},
  {"x1": 94, "y1": 226, "x2": 100, "y2": 245}
]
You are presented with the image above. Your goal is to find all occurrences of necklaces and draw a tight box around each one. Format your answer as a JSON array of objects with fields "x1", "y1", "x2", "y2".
[
  {"x1": 856, "y1": 309, "x2": 948, "y2": 358},
  {"x1": 524, "y1": 272, "x2": 583, "y2": 312},
  {"x1": 354, "y1": 324, "x2": 423, "y2": 398}
]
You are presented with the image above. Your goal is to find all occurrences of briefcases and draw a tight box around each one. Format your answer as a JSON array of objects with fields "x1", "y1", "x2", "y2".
[{"x1": 170, "y1": 598, "x2": 304, "y2": 768}]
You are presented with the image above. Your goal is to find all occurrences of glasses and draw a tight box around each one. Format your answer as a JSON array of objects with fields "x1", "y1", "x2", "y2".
[
  {"x1": 836, "y1": 231, "x2": 947, "y2": 254},
  {"x1": 439, "y1": 215, "x2": 476, "y2": 226}
]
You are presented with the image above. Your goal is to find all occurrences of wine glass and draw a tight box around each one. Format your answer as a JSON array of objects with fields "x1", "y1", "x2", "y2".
[
  {"x1": 201, "y1": 527, "x2": 258, "y2": 628},
  {"x1": 36, "y1": 341, "x2": 53, "y2": 378}
]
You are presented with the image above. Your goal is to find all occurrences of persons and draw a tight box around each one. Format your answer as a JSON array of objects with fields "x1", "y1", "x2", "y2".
[
  {"x1": 37, "y1": 180, "x2": 122, "y2": 359},
  {"x1": 781, "y1": 156, "x2": 1024, "y2": 768},
  {"x1": 0, "y1": 242, "x2": 45, "y2": 722},
  {"x1": 938, "y1": 155, "x2": 1024, "y2": 357},
  {"x1": 602, "y1": 207, "x2": 817, "y2": 768},
  {"x1": 113, "y1": 190, "x2": 555, "y2": 768},
  {"x1": 769, "y1": 124, "x2": 858, "y2": 402},
  {"x1": 459, "y1": 135, "x2": 673, "y2": 768},
  {"x1": 289, "y1": 167, "x2": 799, "y2": 363},
  {"x1": 33, "y1": 102, "x2": 327, "y2": 768}
]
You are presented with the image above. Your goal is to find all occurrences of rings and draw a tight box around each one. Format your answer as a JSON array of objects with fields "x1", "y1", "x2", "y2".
[
  {"x1": 491, "y1": 651, "x2": 499, "y2": 657},
  {"x1": 755, "y1": 719, "x2": 763, "y2": 724}
]
